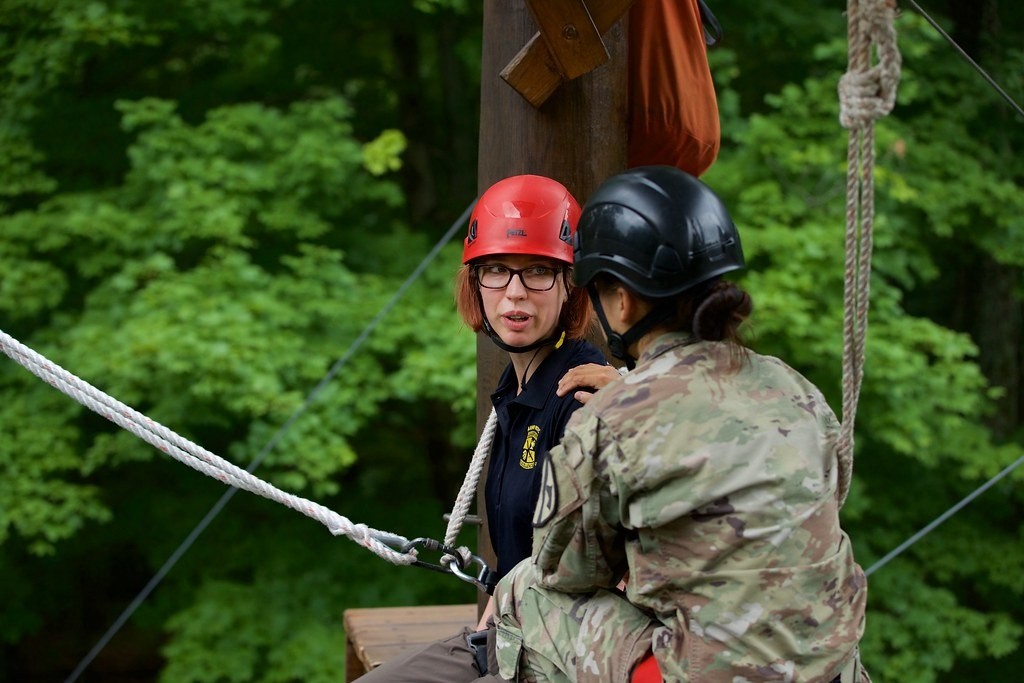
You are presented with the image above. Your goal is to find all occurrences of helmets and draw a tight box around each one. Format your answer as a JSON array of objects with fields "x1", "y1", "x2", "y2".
[
  {"x1": 572, "y1": 165, "x2": 746, "y2": 298},
  {"x1": 463, "y1": 174, "x2": 582, "y2": 265}
]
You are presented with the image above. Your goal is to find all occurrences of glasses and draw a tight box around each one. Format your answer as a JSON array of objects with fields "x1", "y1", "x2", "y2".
[{"x1": 473, "y1": 264, "x2": 570, "y2": 291}]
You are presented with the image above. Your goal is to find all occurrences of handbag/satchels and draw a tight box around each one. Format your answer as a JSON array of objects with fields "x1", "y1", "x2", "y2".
[{"x1": 625, "y1": 0, "x2": 723, "y2": 178}]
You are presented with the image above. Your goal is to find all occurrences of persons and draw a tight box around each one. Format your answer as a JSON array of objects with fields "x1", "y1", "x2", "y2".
[
  {"x1": 350, "y1": 175, "x2": 631, "y2": 683},
  {"x1": 493, "y1": 166, "x2": 867, "y2": 683}
]
